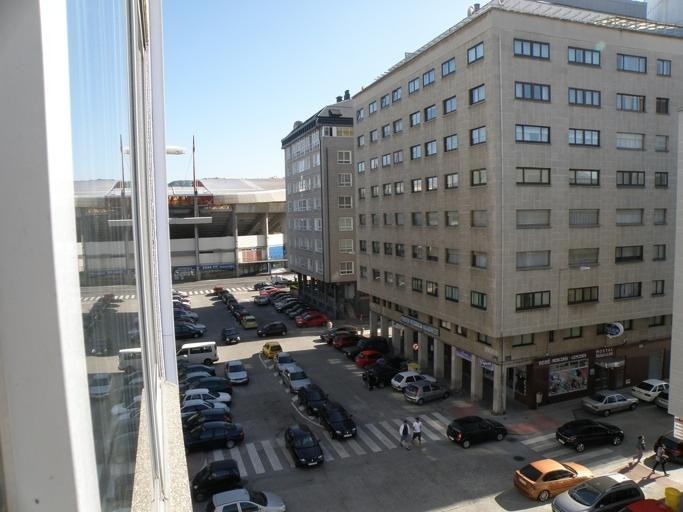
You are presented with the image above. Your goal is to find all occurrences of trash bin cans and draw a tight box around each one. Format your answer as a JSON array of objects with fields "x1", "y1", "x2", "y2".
[
  {"x1": 665, "y1": 487, "x2": 683, "y2": 512},
  {"x1": 408, "y1": 363, "x2": 416, "y2": 371}
]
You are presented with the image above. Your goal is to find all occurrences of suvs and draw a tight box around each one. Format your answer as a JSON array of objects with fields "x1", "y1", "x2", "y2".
[
  {"x1": 554, "y1": 419, "x2": 624, "y2": 453},
  {"x1": 446, "y1": 416, "x2": 507, "y2": 450},
  {"x1": 550, "y1": 471, "x2": 644, "y2": 512},
  {"x1": 652, "y1": 429, "x2": 682, "y2": 464}
]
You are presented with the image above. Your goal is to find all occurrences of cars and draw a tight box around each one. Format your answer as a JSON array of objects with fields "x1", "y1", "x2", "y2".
[
  {"x1": 80, "y1": 287, "x2": 143, "y2": 509},
  {"x1": 616, "y1": 499, "x2": 673, "y2": 512},
  {"x1": 512, "y1": 459, "x2": 594, "y2": 503},
  {"x1": 654, "y1": 387, "x2": 668, "y2": 411},
  {"x1": 630, "y1": 378, "x2": 668, "y2": 402},
  {"x1": 580, "y1": 389, "x2": 639, "y2": 418}
]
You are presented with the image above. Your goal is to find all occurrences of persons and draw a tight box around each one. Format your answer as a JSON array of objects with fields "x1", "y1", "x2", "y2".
[
  {"x1": 652, "y1": 443, "x2": 670, "y2": 476},
  {"x1": 412, "y1": 418, "x2": 423, "y2": 445},
  {"x1": 399, "y1": 420, "x2": 411, "y2": 451},
  {"x1": 633, "y1": 436, "x2": 646, "y2": 462}
]
